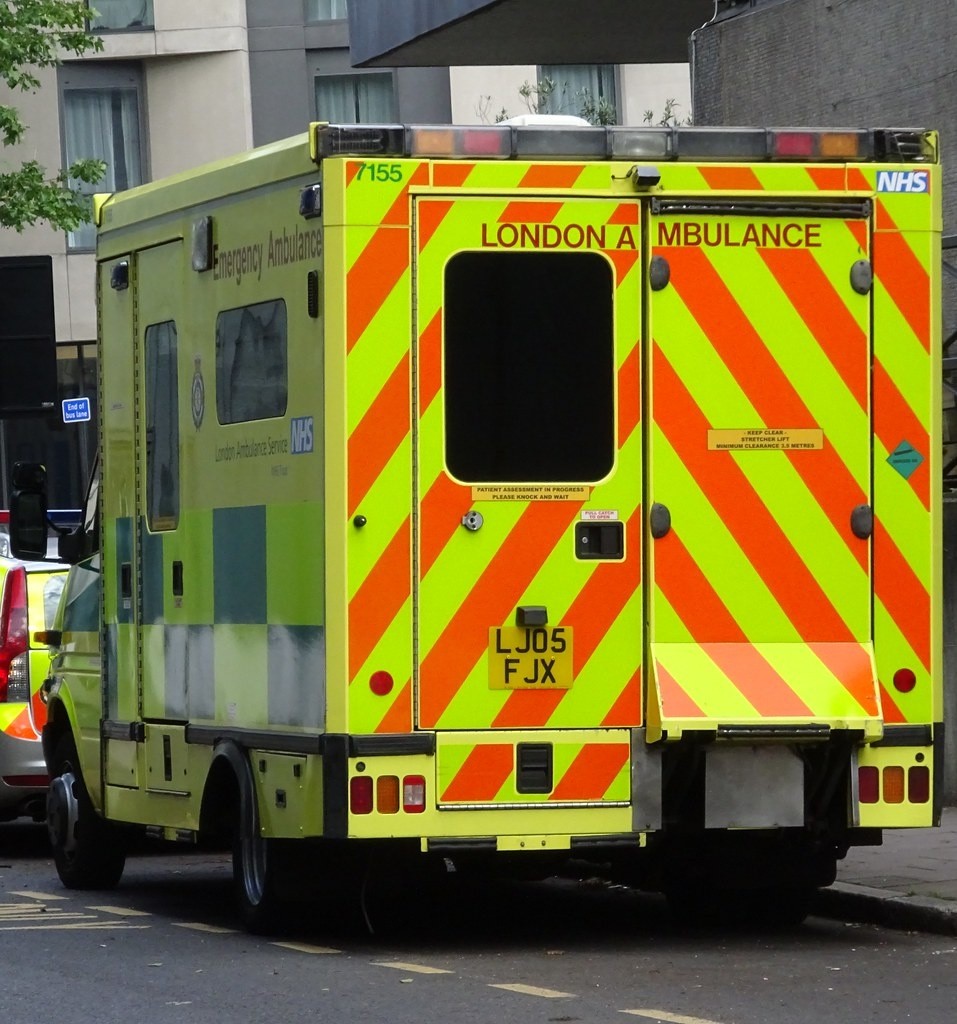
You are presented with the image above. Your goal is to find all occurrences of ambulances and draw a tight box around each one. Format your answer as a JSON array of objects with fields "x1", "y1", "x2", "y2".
[{"x1": 8, "y1": 120, "x2": 947, "y2": 931}]
